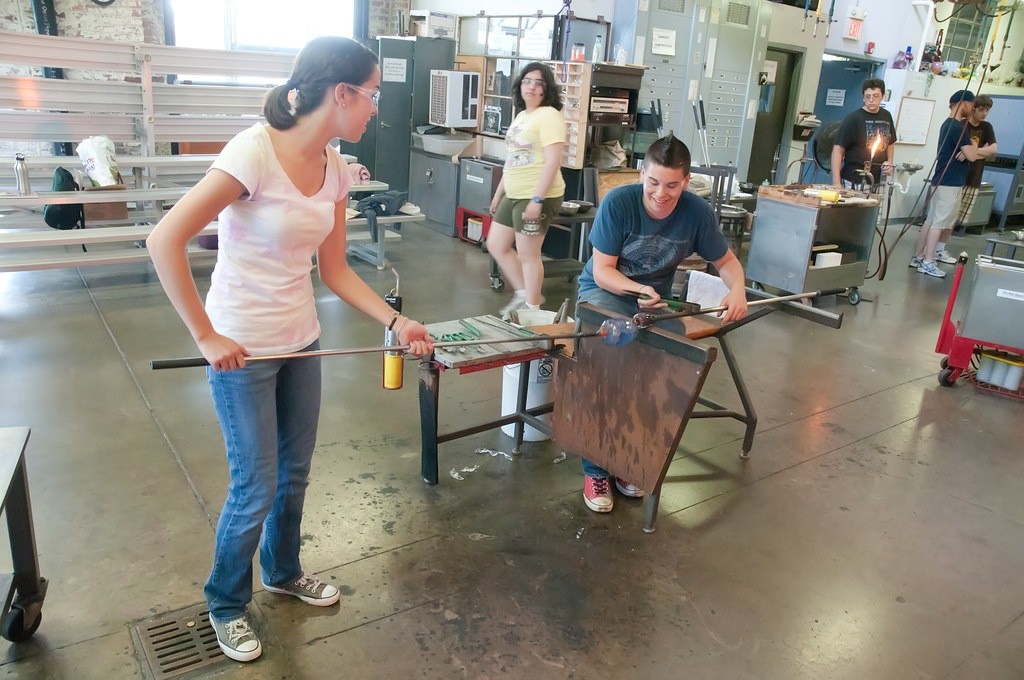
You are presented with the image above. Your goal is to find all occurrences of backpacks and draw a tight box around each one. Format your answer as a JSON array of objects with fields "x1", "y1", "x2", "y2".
[{"x1": 43, "y1": 166, "x2": 87, "y2": 252}]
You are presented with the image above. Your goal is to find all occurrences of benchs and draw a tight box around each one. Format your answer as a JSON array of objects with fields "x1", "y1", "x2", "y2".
[{"x1": 1, "y1": 30, "x2": 426, "y2": 273}]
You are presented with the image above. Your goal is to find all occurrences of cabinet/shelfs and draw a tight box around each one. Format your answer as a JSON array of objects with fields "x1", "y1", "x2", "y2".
[
  {"x1": 352, "y1": 1, "x2": 1024, "y2": 396},
  {"x1": 0, "y1": 425, "x2": 50, "y2": 648}
]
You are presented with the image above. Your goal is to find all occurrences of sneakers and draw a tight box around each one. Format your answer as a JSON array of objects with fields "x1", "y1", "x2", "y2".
[
  {"x1": 208, "y1": 611, "x2": 263, "y2": 662},
  {"x1": 917, "y1": 257, "x2": 947, "y2": 278},
  {"x1": 583, "y1": 474, "x2": 614, "y2": 513},
  {"x1": 262, "y1": 573, "x2": 341, "y2": 606},
  {"x1": 909, "y1": 255, "x2": 924, "y2": 268},
  {"x1": 934, "y1": 249, "x2": 957, "y2": 264},
  {"x1": 614, "y1": 476, "x2": 645, "y2": 498}
]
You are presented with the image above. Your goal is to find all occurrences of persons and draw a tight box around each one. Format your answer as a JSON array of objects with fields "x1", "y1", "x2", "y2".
[
  {"x1": 910, "y1": 90, "x2": 978, "y2": 278},
  {"x1": 147, "y1": 35, "x2": 436, "y2": 660},
  {"x1": 576, "y1": 130, "x2": 747, "y2": 512},
  {"x1": 935, "y1": 94, "x2": 998, "y2": 264},
  {"x1": 488, "y1": 61, "x2": 566, "y2": 316},
  {"x1": 831, "y1": 79, "x2": 898, "y2": 224}
]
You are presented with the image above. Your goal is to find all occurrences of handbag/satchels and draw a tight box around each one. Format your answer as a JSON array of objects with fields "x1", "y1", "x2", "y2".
[{"x1": 76, "y1": 135, "x2": 123, "y2": 188}]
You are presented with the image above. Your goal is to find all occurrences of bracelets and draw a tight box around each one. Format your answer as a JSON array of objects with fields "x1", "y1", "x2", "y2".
[
  {"x1": 493, "y1": 194, "x2": 503, "y2": 196},
  {"x1": 389, "y1": 311, "x2": 408, "y2": 336},
  {"x1": 533, "y1": 196, "x2": 544, "y2": 203}
]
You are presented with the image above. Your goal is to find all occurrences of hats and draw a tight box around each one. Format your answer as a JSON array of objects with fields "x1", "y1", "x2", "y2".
[{"x1": 950, "y1": 90, "x2": 977, "y2": 104}]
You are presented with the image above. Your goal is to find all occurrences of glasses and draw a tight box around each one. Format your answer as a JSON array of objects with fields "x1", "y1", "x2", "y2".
[
  {"x1": 521, "y1": 78, "x2": 546, "y2": 86},
  {"x1": 333, "y1": 81, "x2": 381, "y2": 105}
]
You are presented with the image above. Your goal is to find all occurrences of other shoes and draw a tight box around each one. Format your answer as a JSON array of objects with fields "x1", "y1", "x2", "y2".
[
  {"x1": 498, "y1": 296, "x2": 526, "y2": 317},
  {"x1": 518, "y1": 303, "x2": 542, "y2": 310}
]
